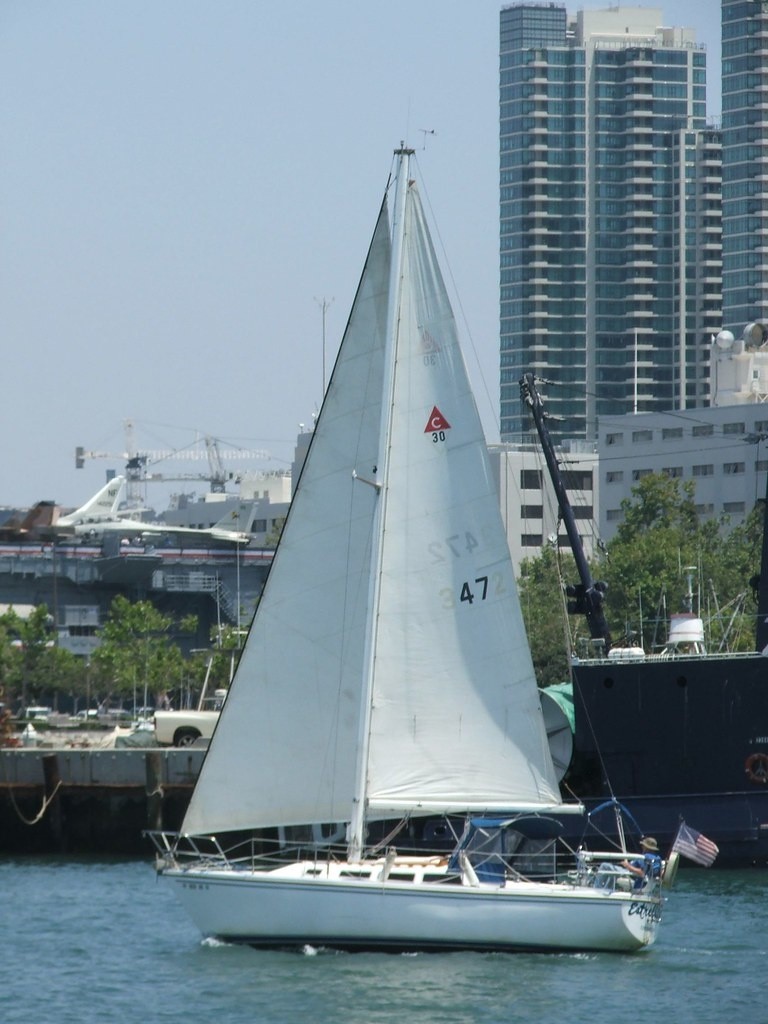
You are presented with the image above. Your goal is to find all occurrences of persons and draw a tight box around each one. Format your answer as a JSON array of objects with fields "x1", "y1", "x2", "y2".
[{"x1": 623, "y1": 838, "x2": 661, "y2": 889}]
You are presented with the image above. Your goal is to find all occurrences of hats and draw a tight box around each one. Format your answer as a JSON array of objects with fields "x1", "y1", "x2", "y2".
[{"x1": 639, "y1": 837, "x2": 659, "y2": 851}]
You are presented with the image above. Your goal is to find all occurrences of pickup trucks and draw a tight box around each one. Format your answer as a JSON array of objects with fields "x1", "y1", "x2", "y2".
[{"x1": 150, "y1": 707, "x2": 220, "y2": 750}]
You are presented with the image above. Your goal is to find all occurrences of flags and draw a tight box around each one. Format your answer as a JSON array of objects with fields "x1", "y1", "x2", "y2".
[{"x1": 672, "y1": 823, "x2": 720, "y2": 867}]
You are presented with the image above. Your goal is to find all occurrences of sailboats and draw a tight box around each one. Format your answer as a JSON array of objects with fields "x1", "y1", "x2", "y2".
[{"x1": 136, "y1": 135, "x2": 670, "y2": 954}]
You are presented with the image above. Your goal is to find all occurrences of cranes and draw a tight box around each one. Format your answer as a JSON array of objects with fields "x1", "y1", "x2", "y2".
[{"x1": 73, "y1": 415, "x2": 267, "y2": 473}]
